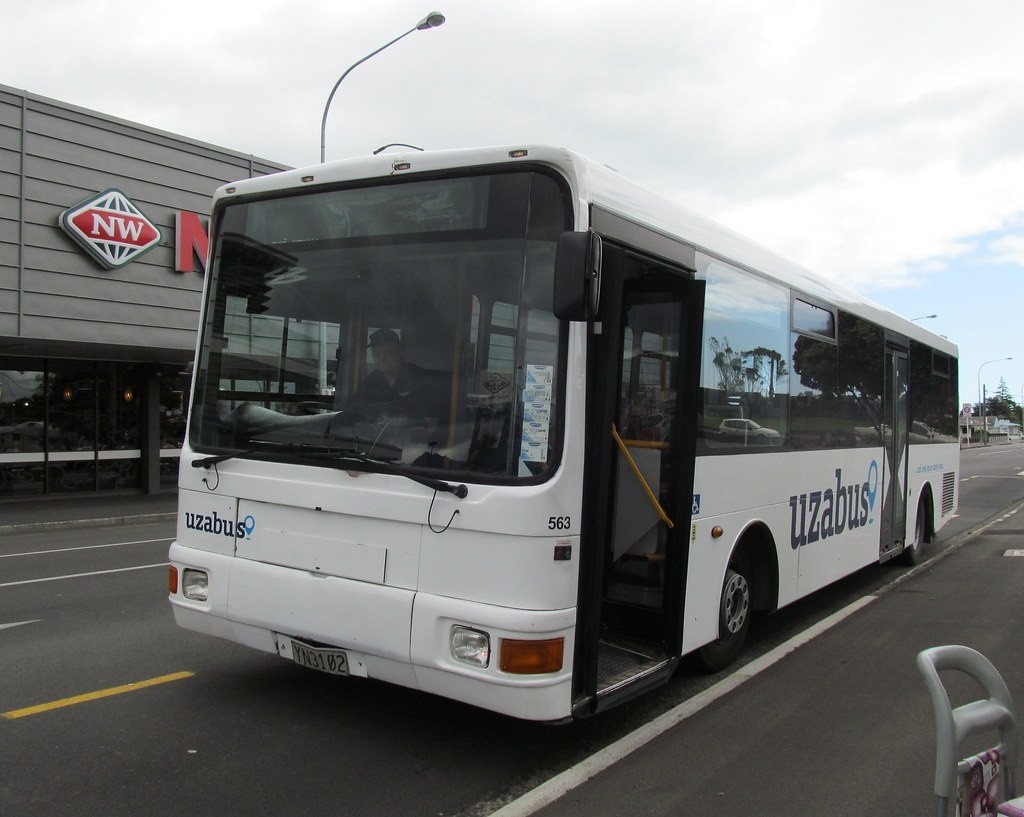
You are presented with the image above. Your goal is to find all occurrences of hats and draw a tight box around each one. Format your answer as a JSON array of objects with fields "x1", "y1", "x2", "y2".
[{"x1": 365, "y1": 328, "x2": 400, "y2": 350}]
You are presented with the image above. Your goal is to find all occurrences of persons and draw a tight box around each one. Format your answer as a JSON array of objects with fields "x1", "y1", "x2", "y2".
[
  {"x1": 621, "y1": 391, "x2": 678, "y2": 441},
  {"x1": 349, "y1": 328, "x2": 430, "y2": 421}
]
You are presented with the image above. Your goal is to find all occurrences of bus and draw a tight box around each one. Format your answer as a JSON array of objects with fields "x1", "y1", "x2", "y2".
[{"x1": 168, "y1": 143, "x2": 963, "y2": 725}]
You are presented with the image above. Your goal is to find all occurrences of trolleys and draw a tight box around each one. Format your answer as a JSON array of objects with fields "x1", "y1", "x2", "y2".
[{"x1": 916, "y1": 642, "x2": 1024, "y2": 817}]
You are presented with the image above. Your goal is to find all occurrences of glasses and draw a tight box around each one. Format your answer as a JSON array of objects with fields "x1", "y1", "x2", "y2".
[{"x1": 371, "y1": 349, "x2": 399, "y2": 357}]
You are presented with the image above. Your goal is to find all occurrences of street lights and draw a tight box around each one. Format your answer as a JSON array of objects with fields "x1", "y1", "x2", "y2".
[
  {"x1": 978, "y1": 357, "x2": 1013, "y2": 445},
  {"x1": 320, "y1": 10, "x2": 445, "y2": 162}
]
